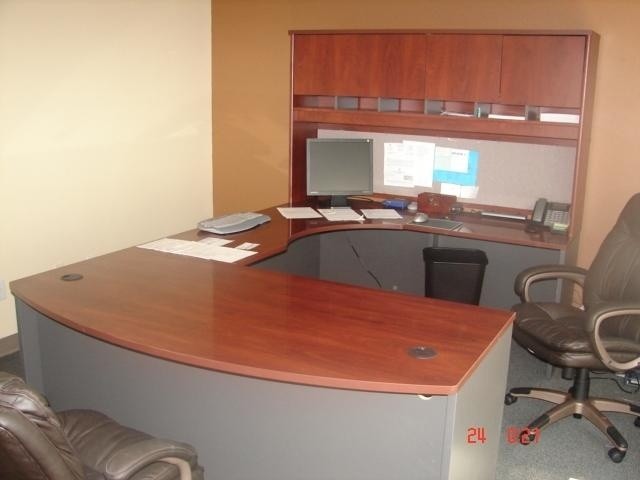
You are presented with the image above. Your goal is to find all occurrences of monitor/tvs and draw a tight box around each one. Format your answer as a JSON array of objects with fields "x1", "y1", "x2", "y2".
[{"x1": 303, "y1": 137, "x2": 376, "y2": 211}]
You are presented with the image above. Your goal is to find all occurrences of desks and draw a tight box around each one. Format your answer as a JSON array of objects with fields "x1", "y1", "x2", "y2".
[{"x1": 10, "y1": 193, "x2": 570, "y2": 480}]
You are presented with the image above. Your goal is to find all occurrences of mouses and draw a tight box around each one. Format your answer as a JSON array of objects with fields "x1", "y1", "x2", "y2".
[{"x1": 412, "y1": 213, "x2": 429, "y2": 223}]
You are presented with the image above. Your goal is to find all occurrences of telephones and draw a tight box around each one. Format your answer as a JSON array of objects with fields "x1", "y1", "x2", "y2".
[{"x1": 525, "y1": 197, "x2": 571, "y2": 236}]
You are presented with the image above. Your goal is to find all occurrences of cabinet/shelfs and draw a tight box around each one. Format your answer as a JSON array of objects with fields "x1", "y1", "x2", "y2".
[{"x1": 288, "y1": 26, "x2": 596, "y2": 233}]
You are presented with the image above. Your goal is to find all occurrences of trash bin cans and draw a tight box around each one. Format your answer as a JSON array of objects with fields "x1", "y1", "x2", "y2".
[{"x1": 422, "y1": 246, "x2": 489, "y2": 306}]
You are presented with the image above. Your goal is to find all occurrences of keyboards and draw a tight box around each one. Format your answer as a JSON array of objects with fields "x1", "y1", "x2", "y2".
[{"x1": 197, "y1": 212, "x2": 271, "y2": 235}]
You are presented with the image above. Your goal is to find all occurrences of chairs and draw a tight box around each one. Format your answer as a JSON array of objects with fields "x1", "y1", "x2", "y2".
[
  {"x1": 502, "y1": 191, "x2": 640, "y2": 464},
  {"x1": 1, "y1": 370, "x2": 205, "y2": 480}
]
